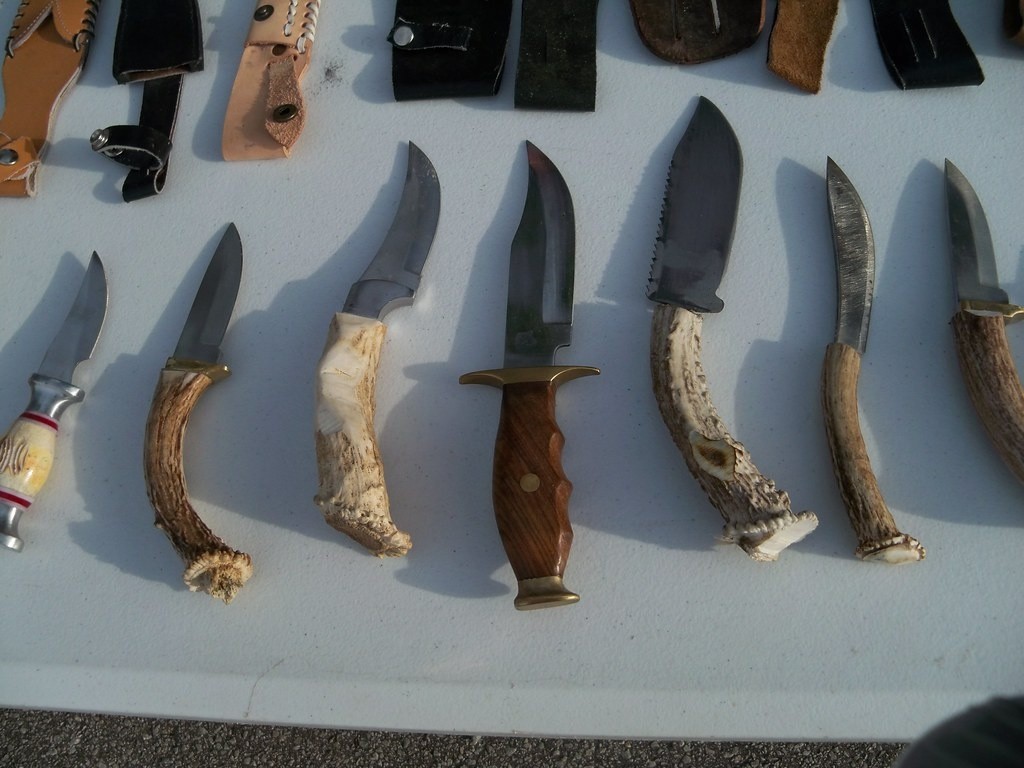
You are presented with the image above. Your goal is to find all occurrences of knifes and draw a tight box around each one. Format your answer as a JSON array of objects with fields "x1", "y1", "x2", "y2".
[
  {"x1": 943, "y1": 156, "x2": 1024, "y2": 486},
  {"x1": 317, "y1": 142, "x2": 441, "y2": 558},
  {"x1": 144, "y1": 223, "x2": 255, "y2": 603},
  {"x1": 459, "y1": 140, "x2": 601, "y2": 611},
  {"x1": 645, "y1": 96, "x2": 820, "y2": 560},
  {"x1": 1, "y1": 251, "x2": 110, "y2": 553},
  {"x1": 821, "y1": 153, "x2": 927, "y2": 564}
]
